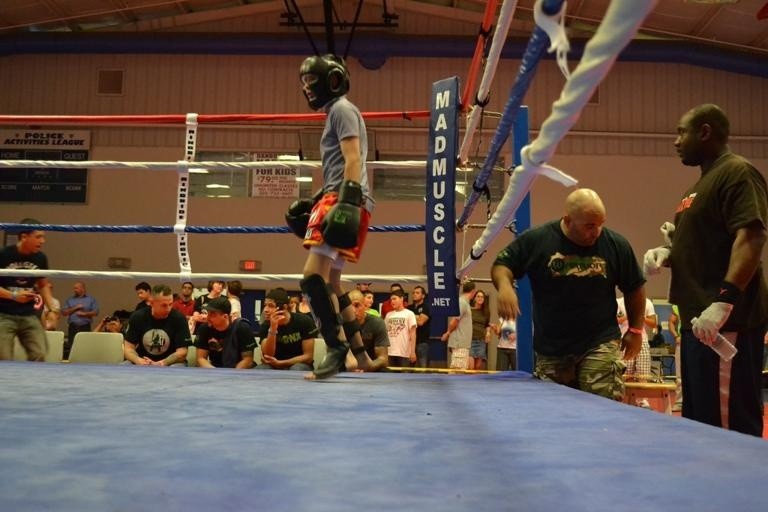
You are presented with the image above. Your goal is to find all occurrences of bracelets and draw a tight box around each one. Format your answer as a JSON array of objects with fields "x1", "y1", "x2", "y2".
[
  {"x1": 12, "y1": 290, "x2": 16, "y2": 301},
  {"x1": 673, "y1": 334, "x2": 679, "y2": 339},
  {"x1": 627, "y1": 327, "x2": 643, "y2": 334},
  {"x1": 712, "y1": 280, "x2": 742, "y2": 305}
]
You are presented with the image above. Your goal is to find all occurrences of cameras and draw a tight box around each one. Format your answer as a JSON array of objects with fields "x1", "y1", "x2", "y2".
[{"x1": 105, "y1": 315, "x2": 117, "y2": 322}]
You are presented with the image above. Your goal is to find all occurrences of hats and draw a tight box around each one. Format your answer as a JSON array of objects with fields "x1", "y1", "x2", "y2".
[
  {"x1": 356, "y1": 280, "x2": 371, "y2": 284},
  {"x1": 199, "y1": 297, "x2": 230, "y2": 313}
]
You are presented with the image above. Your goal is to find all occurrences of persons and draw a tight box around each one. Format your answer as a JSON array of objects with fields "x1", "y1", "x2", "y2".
[
  {"x1": 94, "y1": 281, "x2": 391, "y2": 370},
  {"x1": 382, "y1": 284, "x2": 430, "y2": 367},
  {"x1": 668, "y1": 305, "x2": 683, "y2": 411},
  {"x1": 643, "y1": 104, "x2": 768, "y2": 438},
  {"x1": 616, "y1": 295, "x2": 656, "y2": 382},
  {"x1": 440, "y1": 282, "x2": 516, "y2": 374},
  {"x1": 491, "y1": 188, "x2": 647, "y2": 402},
  {"x1": 41, "y1": 282, "x2": 61, "y2": 331},
  {"x1": 285, "y1": 53, "x2": 376, "y2": 381},
  {"x1": 61, "y1": 280, "x2": 99, "y2": 360},
  {"x1": 0, "y1": 218, "x2": 60, "y2": 361}
]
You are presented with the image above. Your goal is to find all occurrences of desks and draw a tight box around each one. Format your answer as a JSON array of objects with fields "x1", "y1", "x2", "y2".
[
  {"x1": 651, "y1": 354, "x2": 675, "y2": 375},
  {"x1": 625, "y1": 382, "x2": 677, "y2": 415}
]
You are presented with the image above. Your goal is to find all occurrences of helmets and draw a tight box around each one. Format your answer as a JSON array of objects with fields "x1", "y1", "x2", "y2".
[{"x1": 299, "y1": 53, "x2": 349, "y2": 111}]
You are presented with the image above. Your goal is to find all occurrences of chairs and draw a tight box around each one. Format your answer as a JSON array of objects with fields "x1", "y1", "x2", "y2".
[
  {"x1": 13, "y1": 330, "x2": 64, "y2": 362},
  {"x1": 69, "y1": 332, "x2": 124, "y2": 363}
]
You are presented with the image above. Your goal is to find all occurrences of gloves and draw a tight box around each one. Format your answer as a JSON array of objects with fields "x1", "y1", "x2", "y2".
[
  {"x1": 285, "y1": 187, "x2": 324, "y2": 238},
  {"x1": 319, "y1": 179, "x2": 363, "y2": 249}
]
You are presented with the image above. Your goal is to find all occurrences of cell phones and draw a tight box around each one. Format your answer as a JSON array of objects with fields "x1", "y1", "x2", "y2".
[{"x1": 277, "y1": 302, "x2": 283, "y2": 315}]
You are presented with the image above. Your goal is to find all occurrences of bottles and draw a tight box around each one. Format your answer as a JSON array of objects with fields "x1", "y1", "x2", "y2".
[{"x1": 691, "y1": 316, "x2": 738, "y2": 362}]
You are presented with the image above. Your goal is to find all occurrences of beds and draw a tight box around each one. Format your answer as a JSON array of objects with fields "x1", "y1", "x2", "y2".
[{"x1": 186, "y1": 335, "x2": 327, "y2": 369}]
